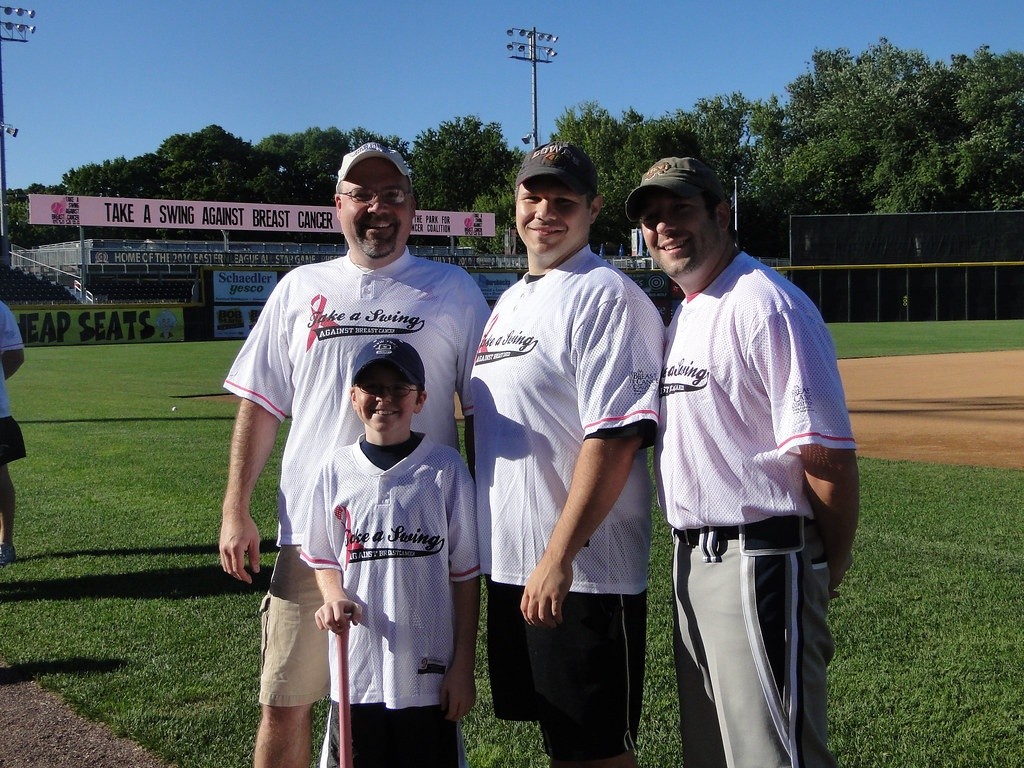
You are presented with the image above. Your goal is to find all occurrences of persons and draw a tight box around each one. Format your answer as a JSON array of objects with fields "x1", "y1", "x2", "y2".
[
  {"x1": 625, "y1": 153, "x2": 859, "y2": 768},
  {"x1": 218, "y1": 140, "x2": 492, "y2": 767},
  {"x1": 0, "y1": 298, "x2": 27, "y2": 568},
  {"x1": 297, "y1": 336, "x2": 480, "y2": 768},
  {"x1": 467, "y1": 140, "x2": 665, "y2": 768}
]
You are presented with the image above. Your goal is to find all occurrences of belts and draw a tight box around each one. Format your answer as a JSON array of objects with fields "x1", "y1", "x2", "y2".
[{"x1": 677, "y1": 527, "x2": 739, "y2": 543}]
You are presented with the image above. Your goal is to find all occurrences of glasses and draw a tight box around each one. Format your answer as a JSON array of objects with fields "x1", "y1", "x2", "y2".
[
  {"x1": 353, "y1": 382, "x2": 419, "y2": 396},
  {"x1": 336, "y1": 187, "x2": 411, "y2": 204}
]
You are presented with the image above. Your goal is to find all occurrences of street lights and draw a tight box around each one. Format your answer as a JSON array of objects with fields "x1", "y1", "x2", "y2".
[
  {"x1": 506, "y1": 26, "x2": 558, "y2": 149},
  {"x1": 0, "y1": 6, "x2": 36, "y2": 270}
]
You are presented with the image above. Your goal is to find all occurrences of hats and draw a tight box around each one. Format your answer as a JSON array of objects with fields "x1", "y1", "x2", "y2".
[
  {"x1": 352, "y1": 337, "x2": 425, "y2": 388},
  {"x1": 336, "y1": 143, "x2": 412, "y2": 191},
  {"x1": 626, "y1": 157, "x2": 722, "y2": 222},
  {"x1": 516, "y1": 141, "x2": 597, "y2": 196}
]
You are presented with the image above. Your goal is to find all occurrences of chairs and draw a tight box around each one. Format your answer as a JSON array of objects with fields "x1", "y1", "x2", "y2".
[{"x1": 0, "y1": 263, "x2": 195, "y2": 305}]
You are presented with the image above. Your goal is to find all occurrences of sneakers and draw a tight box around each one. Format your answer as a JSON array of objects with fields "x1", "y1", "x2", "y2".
[{"x1": 0, "y1": 544, "x2": 14, "y2": 567}]
